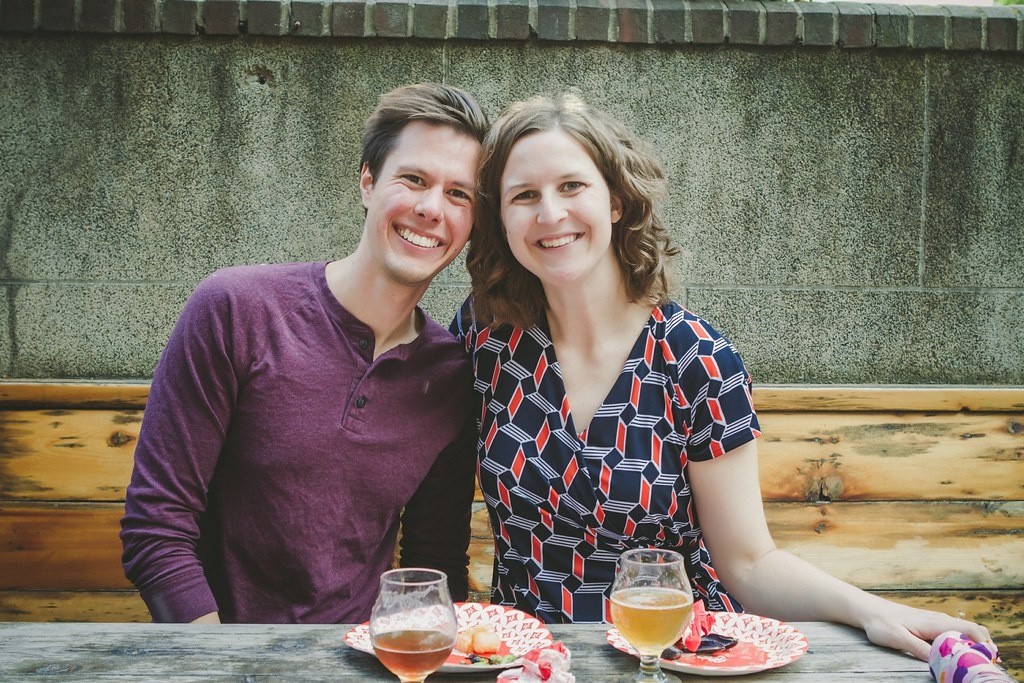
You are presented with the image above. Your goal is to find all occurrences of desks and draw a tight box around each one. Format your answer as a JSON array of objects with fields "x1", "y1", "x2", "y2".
[{"x1": 0, "y1": 620, "x2": 934, "y2": 682}]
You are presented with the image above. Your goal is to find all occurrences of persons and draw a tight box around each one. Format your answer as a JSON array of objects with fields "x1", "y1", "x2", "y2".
[
  {"x1": 119, "y1": 81, "x2": 492, "y2": 624},
  {"x1": 446, "y1": 95, "x2": 1002, "y2": 664}
]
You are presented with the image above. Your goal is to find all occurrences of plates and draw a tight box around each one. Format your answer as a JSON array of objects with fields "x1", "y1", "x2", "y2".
[
  {"x1": 605, "y1": 612, "x2": 810, "y2": 676},
  {"x1": 342, "y1": 602, "x2": 555, "y2": 674}
]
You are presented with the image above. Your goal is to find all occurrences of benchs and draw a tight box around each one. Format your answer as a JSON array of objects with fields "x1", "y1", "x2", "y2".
[{"x1": 0, "y1": 378, "x2": 1024, "y2": 683}]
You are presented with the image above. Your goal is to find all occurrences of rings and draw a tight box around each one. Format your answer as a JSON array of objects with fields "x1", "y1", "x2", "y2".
[{"x1": 956, "y1": 611, "x2": 965, "y2": 619}]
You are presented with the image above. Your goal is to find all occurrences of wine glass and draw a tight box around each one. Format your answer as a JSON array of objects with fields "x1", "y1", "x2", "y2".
[
  {"x1": 369, "y1": 568, "x2": 457, "y2": 683},
  {"x1": 610, "y1": 547, "x2": 694, "y2": 683}
]
